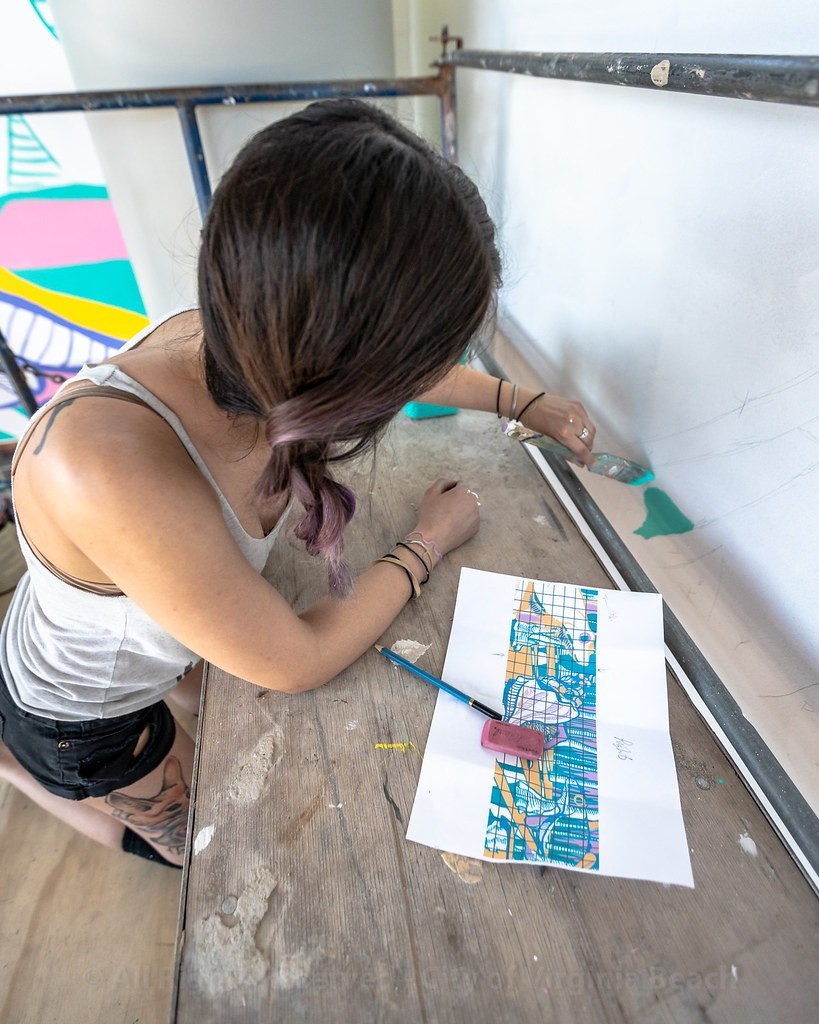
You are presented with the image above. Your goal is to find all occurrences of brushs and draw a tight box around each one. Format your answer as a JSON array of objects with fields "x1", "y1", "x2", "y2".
[{"x1": 500, "y1": 420, "x2": 654, "y2": 487}]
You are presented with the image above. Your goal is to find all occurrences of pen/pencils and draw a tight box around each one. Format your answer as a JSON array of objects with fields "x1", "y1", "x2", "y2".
[{"x1": 370, "y1": 642, "x2": 503, "y2": 722}]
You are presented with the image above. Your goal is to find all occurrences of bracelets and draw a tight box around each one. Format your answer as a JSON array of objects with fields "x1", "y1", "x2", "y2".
[
  {"x1": 371, "y1": 530, "x2": 443, "y2": 602},
  {"x1": 496, "y1": 376, "x2": 545, "y2": 427}
]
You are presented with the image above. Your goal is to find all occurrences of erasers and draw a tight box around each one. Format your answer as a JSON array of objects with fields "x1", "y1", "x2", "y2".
[{"x1": 482, "y1": 718, "x2": 545, "y2": 761}]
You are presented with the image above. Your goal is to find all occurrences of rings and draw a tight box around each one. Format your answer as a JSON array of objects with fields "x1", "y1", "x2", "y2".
[{"x1": 579, "y1": 426, "x2": 589, "y2": 440}]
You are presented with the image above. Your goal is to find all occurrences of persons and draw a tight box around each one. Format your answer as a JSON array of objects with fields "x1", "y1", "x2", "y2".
[{"x1": 0, "y1": 99, "x2": 598, "y2": 873}]
